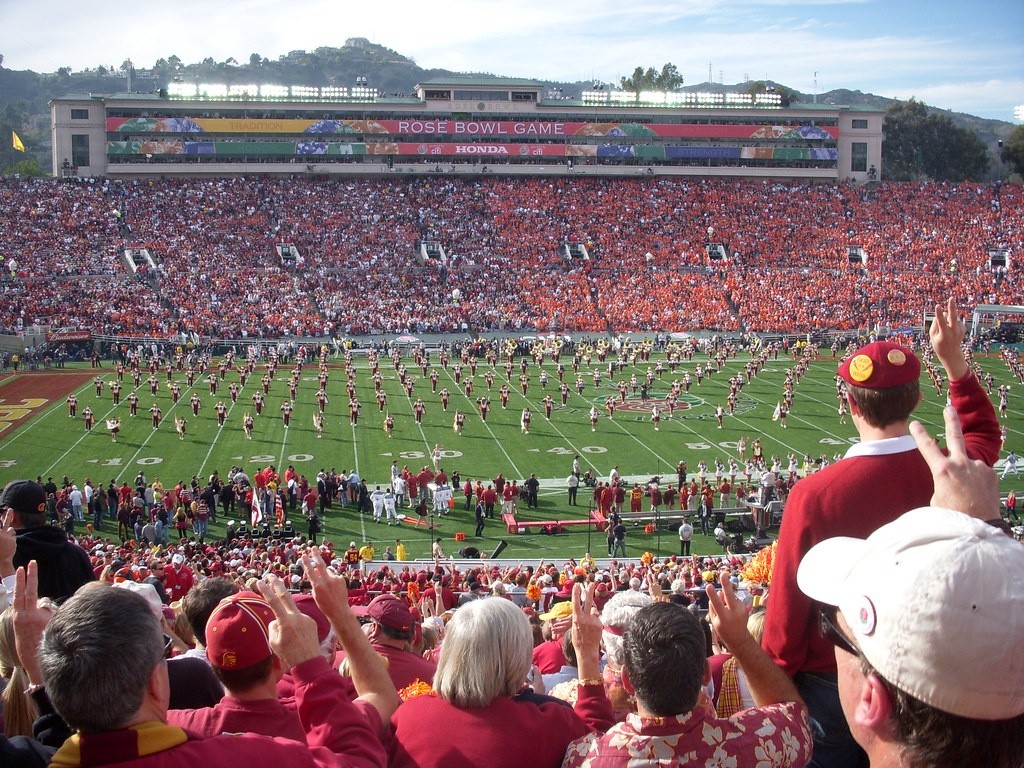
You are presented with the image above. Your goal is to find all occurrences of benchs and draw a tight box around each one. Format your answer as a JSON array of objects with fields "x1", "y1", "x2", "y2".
[
  {"x1": 503, "y1": 514, "x2": 517, "y2": 534},
  {"x1": 590, "y1": 510, "x2": 608, "y2": 531},
  {"x1": 517, "y1": 521, "x2": 558, "y2": 534},
  {"x1": 609, "y1": 507, "x2": 750, "y2": 529},
  {"x1": 558, "y1": 519, "x2": 599, "y2": 532}
]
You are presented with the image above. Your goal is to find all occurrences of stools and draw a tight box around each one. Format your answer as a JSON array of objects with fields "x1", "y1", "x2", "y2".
[
  {"x1": 644, "y1": 525, "x2": 654, "y2": 533},
  {"x1": 456, "y1": 533, "x2": 465, "y2": 541}
]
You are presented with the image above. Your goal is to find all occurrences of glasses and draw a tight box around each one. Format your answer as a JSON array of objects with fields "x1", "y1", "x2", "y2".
[
  {"x1": 815, "y1": 607, "x2": 860, "y2": 661},
  {"x1": 156, "y1": 567, "x2": 164, "y2": 571},
  {"x1": 158, "y1": 633, "x2": 175, "y2": 660}
]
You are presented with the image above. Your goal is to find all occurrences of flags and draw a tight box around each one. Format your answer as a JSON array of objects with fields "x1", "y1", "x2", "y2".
[
  {"x1": 275, "y1": 492, "x2": 286, "y2": 530},
  {"x1": 13, "y1": 131, "x2": 25, "y2": 152},
  {"x1": 250, "y1": 487, "x2": 263, "y2": 527}
]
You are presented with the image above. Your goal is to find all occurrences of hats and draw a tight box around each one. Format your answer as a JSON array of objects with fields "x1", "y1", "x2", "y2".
[
  {"x1": 291, "y1": 574, "x2": 301, "y2": 582},
  {"x1": 147, "y1": 535, "x2": 761, "y2": 614},
  {"x1": 367, "y1": 594, "x2": 412, "y2": 631},
  {"x1": 0, "y1": 479, "x2": 46, "y2": 514},
  {"x1": 172, "y1": 553, "x2": 184, "y2": 565},
  {"x1": 409, "y1": 606, "x2": 420, "y2": 618},
  {"x1": 795, "y1": 506, "x2": 1023, "y2": 720},
  {"x1": 206, "y1": 590, "x2": 280, "y2": 669},
  {"x1": 837, "y1": 340, "x2": 921, "y2": 388},
  {"x1": 539, "y1": 601, "x2": 574, "y2": 621}
]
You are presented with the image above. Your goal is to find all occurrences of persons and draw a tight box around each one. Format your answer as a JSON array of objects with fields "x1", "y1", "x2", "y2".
[{"x1": 0, "y1": 92, "x2": 1024, "y2": 768}]
